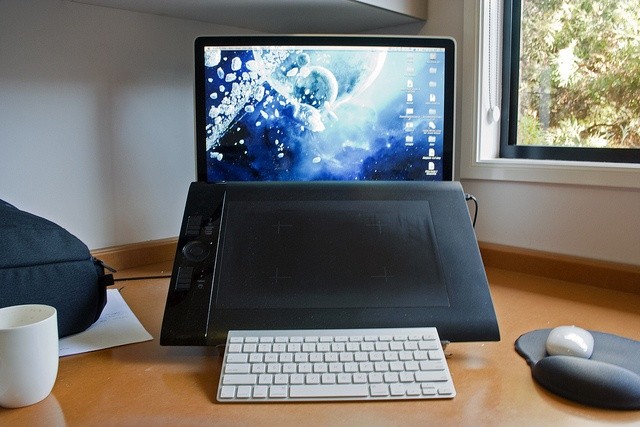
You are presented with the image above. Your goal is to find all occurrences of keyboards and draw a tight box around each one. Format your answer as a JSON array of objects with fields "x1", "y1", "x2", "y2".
[{"x1": 216, "y1": 326, "x2": 457, "y2": 403}]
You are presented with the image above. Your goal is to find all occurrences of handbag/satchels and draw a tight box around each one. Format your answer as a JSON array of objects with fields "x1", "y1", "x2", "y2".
[{"x1": 1, "y1": 200, "x2": 118, "y2": 339}]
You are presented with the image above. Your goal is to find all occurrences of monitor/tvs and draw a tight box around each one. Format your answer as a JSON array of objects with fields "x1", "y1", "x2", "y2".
[{"x1": 194, "y1": 34, "x2": 457, "y2": 183}]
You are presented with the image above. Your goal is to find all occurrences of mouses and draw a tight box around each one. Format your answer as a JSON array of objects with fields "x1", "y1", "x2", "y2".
[{"x1": 545, "y1": 324, "x2": 594, "y2": 359}]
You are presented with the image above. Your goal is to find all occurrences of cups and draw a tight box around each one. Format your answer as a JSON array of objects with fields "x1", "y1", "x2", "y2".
[{"x1": 0, "y1": 304, "x2": 59, "y2": 408}]
